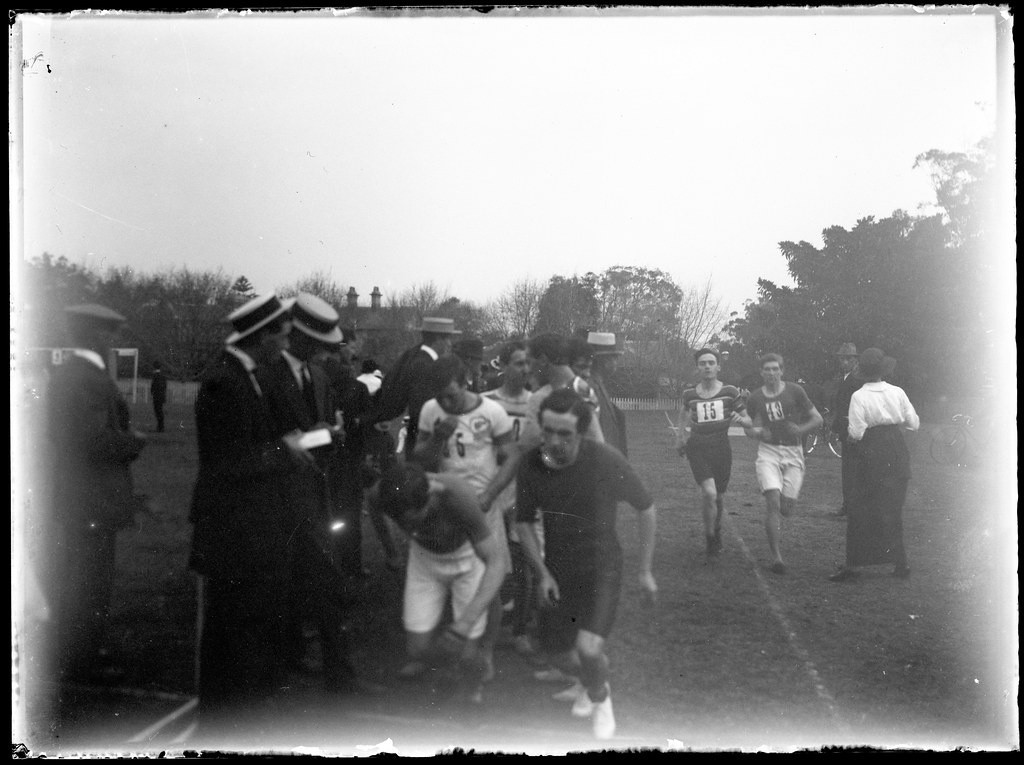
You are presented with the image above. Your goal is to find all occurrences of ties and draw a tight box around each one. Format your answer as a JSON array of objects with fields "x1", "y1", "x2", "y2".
[
  {"x1": 299, "y1": 365, "x2": 316, "y2": 427},
  {"x1": 841, "y1": 374, "x2": 844, "y2": 383},
  {"x1": 253, "y1": 369, "x2": 271, "y2": 410}
]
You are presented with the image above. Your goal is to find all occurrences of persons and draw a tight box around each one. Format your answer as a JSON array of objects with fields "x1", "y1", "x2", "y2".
[
  {"x1": 51, "y1": 305, "x2": 144, "y2": 714},
  {"x1": 828, "y1": 342, "x2": 920, "y2": 581},
  {"x1": 191, "y1": 292, "x2": 658, "y2": 744},
  {"x1": 674, "y1": 349, "x2": 753, "y2": 562},
  {"x1": 742, "y1": 354, "x2": 823, "y2": 573},
  {"x1": 150, "y1": 362, "x2": 167, "y2": 432}
]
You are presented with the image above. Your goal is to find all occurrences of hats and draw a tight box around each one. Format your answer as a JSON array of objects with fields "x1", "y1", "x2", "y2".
[
  {"x1": 222, "y1": 295, "x2": 297, "y2": 344},
  {"x1": 64, "y1": 303, "x2": 125, "y2": 335},
  {"x1": 851, "y1": 347, "x2": 896, "y2": 381},
  {"x1": 491, "y1": 356, "x2": 502, "y2": 368},
  {"x1": 289, "y1": 293, "x2": 344, "y2": 343},
  {"x1": 836, "y1": 342, "x2": 860, "y2": 356},
  {"x1": 361, "y1": 359, "x2": 377, "y2": 372},
  {"x1": 585, "y1": 331, "x2": 625, "y2": 356},
  {"x1": 417, "y1": 316, "x2": 464, "y2": 335}
]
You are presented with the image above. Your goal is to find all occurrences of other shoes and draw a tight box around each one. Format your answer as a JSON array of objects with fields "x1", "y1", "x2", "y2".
[
  {"x1": 549, "y1": 679, "x2": 587, "y2": 701},
  {"x1": 534, "y1": 669, "x2": 578, "y2": 685},
  {"x1": 321, "y1": 655, "x2": 355, "y2": 676},
  {"x1": 511, "y1": 634, "x2": 534, "y2": 657},
  {"x1": 891, "y1": 564, "x2": 910, "y2": 578},
  {"x1": 708, "y1": 545, "x2": 719, "y2": 564},
  {"x1": 474, "y1": 649, "x2": 493, "y2": 681},
  {"x1": 571, "y1": 685, "x2": 610, "y2": 717},
  {"x1": 399, "y1": 651, "x2": 439, "y2": 677},
  {"x1": 772, "y1": 561, "x2": 787, "y2": 573},
  {"x1": 713, "y1": 529, "x2": 722, "y2": 549},
  {"x1": 590, "y1": 699, "x2": 616, "y2": 727},
  {"x1": 827, "y1": 563, "x2": 864, "y2": 581}
]
